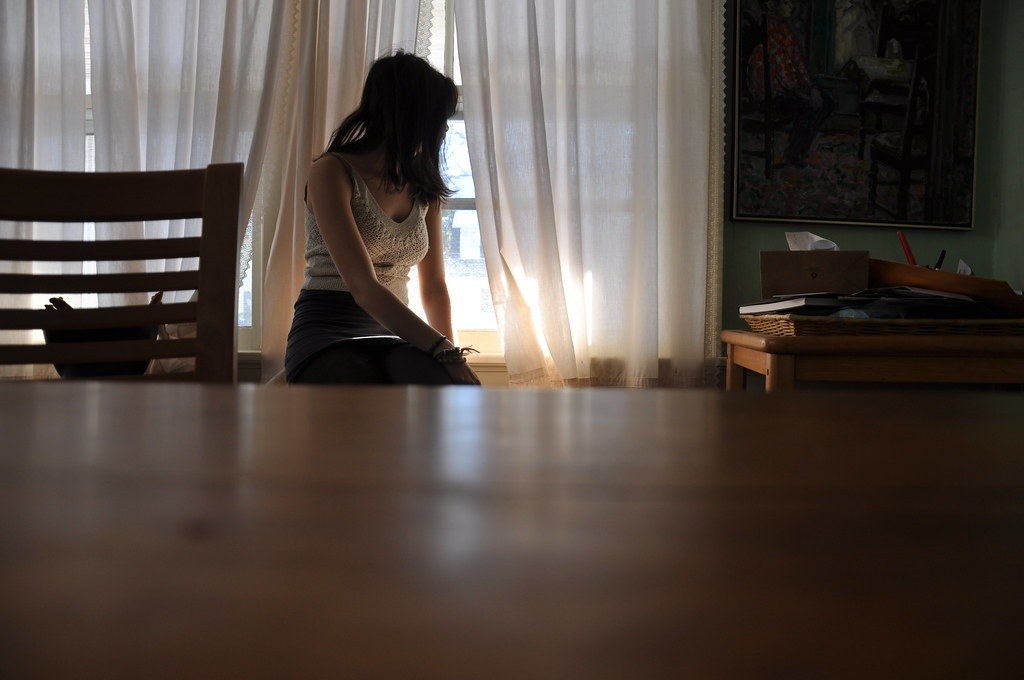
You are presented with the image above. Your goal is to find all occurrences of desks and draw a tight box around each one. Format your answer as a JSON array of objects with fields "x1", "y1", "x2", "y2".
[
  {"x1": 721, "y1": 328, "x2": 1024, "y2": 393},
  {"x1": 0, "y1": 383, "x2": 1024, "y2": 680}
]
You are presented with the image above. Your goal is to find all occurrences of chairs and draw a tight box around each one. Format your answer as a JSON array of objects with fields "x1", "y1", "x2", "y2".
[{"x1": 0, "y1": 162, "x2": 244, "y2": 382}]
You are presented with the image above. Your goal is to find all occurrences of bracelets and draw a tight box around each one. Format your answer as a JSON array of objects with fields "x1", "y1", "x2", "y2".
[
  {"x1": 427, "y1": 336, "x2": 446, "y2": 356},
  {"x1": 435, "y1": 346, "x2": 480, "y2": 364}
]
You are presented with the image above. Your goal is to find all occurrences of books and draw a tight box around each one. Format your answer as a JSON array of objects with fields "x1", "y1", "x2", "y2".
[{"x1": 737, "y1": 296, "x2": 846, "y2": 316}]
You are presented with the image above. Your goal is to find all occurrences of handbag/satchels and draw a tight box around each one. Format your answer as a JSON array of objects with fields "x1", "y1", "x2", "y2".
[{"x1": 43, "y1": 290, "x2": 168, "y2": 379}]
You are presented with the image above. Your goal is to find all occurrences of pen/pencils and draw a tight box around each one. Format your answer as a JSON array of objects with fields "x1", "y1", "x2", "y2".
[
  {"x1": 934, "y1": 246, "x2": 948, "y2": 270},
  {"x1": 895, "y1": 230, "x2": 917, "y2": 266}
]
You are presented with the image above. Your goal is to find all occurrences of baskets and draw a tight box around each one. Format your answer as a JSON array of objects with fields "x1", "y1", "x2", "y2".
[{"x1": 739, "y1": 314, "x2": 1024, "y2": 337}]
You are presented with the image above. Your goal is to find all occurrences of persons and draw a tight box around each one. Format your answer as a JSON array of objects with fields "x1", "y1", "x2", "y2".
[{"x1": 285, "y1": 49, "x2": 483, "y2": 386}]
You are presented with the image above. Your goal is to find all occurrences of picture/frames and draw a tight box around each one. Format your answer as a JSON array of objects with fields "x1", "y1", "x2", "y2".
[{"x1": 729, "y1": 0, "x2": 984, "y2": 232}]
